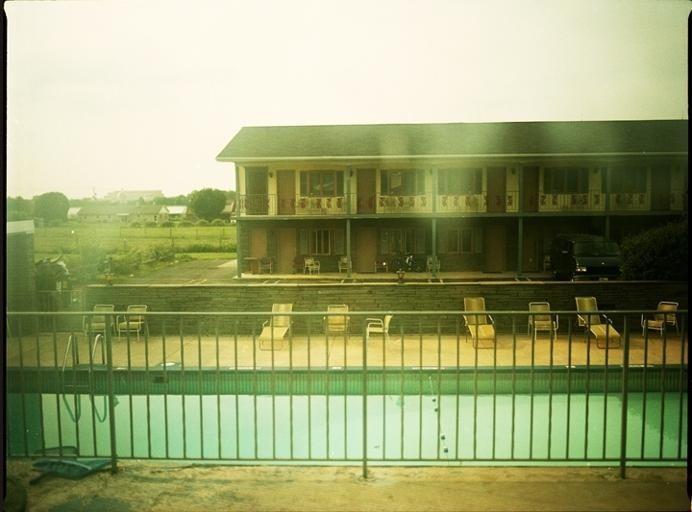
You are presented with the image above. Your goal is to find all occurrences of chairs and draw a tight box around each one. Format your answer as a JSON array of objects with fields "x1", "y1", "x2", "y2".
[
  {"x1": 116, "y1": 304, "x2": 149, "y2": 342},
  {"x1": 366, "y1": 314, "x2": 393, "y2": 340},
  {"x1": 258, "y1": 303, "x2": 294, "y2": 351},
  {"x1": 258, "y1": 254, "x2": 442, "y2": 275},
  {"x1": 576, "y1": 296, "x2": 622, "y2": 349},
  {"x1": 85, "y1": 304, "x2": 116, "y2": 337},
  {"x1": 543, "y1": 255, "x2": 551, "y2": 272},
  {"x1": 323, "y1": 304, "x2": 351, "y2": 345},
  {"x1": 641, "y1": 301, "x2": 679, "y2": 337},
  {"x1": 528, "y1": 301, "x2": 559, "y2": 341},
  {"x1": 462, "y1": 297, "x2": 495, "y2": 349}
]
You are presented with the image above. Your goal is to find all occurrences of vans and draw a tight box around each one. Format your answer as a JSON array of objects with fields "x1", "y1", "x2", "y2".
[{"x1": 548, "y1": 229, "x2": 629, "y2": 282}]
